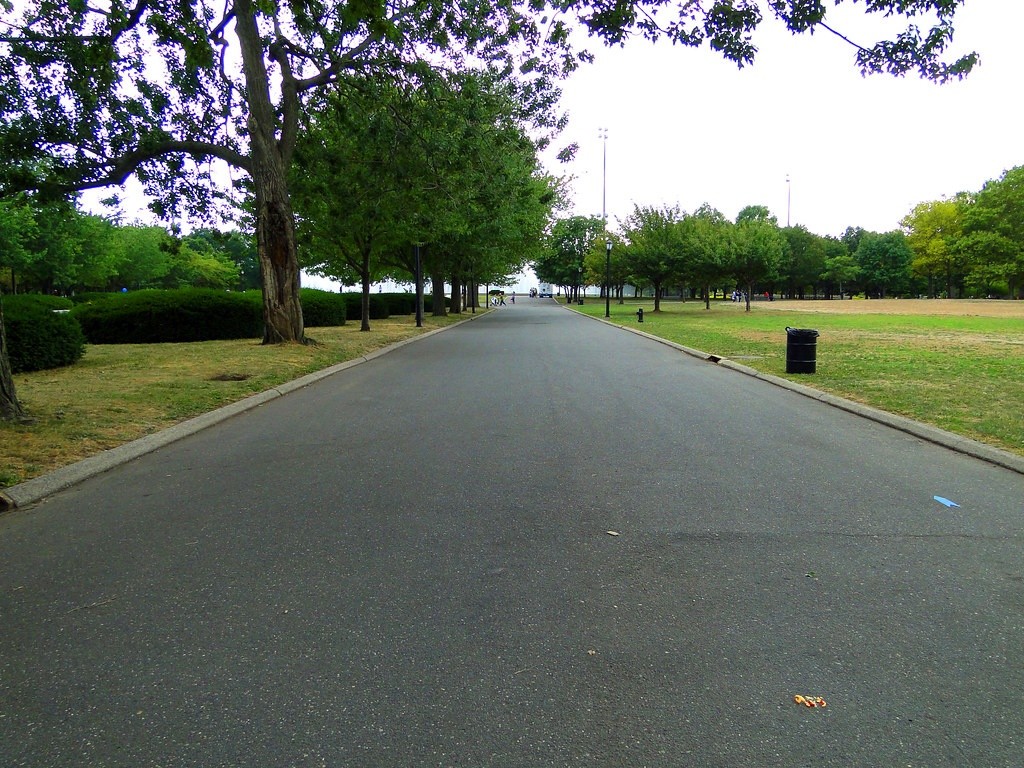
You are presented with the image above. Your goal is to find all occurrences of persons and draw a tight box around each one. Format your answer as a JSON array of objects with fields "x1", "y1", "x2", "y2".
[
  {"x1": 744, "y1": 293, "x2": 750, "y2": 309},
  {"x1": 379, "y1": 284, "x2": 382, "y2": 293},
  {"x1": 764, "y1": 292, "x2": 769, "y2": 300},
  {"x1": 754, "y1": 292, "x2": 759, "y2": 300},
  {"x1": 430, "y1": 291, "x2": 434, "y2": 296},
  {"x1": 497, "y1": 293, "x2": 506, "y2": 306},
  {"x1": 736, "y1": 290, "x2": 741, "y2": 296},
  {"x1": 529, "y1": 287, "x2": 537, "y2": 298},
  {"x1": 339, "y1": 285, "x2": 342, "y2": 294},
  {"x1": 510, "y1": 291, "x2": 515, "y2": 304},
  {"x1": 403, "y1": 286, "x2": 412, "y2": 293}
]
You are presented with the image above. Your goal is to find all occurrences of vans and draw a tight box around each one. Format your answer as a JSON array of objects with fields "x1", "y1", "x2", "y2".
[{"x1": 538, "y1": 283, "x2": 553, "y2": 299}]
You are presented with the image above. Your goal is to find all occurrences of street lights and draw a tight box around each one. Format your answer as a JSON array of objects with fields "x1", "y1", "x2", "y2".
[
  {"x1": 785, "y1": 172, "x2": 792, "y2": 228},
  {"x1": 598, "y1": 127, "x2": 609, "y2": 248},
  {"x1": 603, "y1": 239, "x2": 614, "y2": 319}
]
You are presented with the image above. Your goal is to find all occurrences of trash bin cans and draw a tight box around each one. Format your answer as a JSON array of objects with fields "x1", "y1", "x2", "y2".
[
  {"x1": 557, "y1": 293, "x2": 559, "y2": 296},
  {"x1": 785, "y1": 327, "x2": 819, "y2": 373},
  {"x1": 579, "y1": 299, "x2": 583, "y2": 305},
  {"x1": 567, "y1": 297, "x2": 571, "y2": 303}
]
